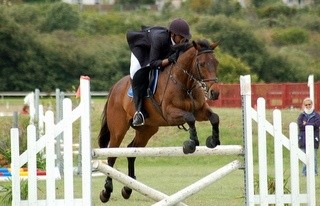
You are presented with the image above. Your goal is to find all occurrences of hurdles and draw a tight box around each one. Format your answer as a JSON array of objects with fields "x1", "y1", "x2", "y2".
[{"x1": 9, "y1": 73, "x2": 317, "y2": 206}]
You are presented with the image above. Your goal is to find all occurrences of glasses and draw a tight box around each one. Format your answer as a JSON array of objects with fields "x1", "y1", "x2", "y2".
[
  {"x1": 181, "y1": 36, "x2": 183, "y2": 39},
  {"x1": 305, "y1": 104, "x2": 311, "y2": 106}
]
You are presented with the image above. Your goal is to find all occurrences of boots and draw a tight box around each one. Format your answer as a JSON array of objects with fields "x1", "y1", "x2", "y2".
[{"x1": 130, "y1": 66, "x2": 149, "y2": 124}]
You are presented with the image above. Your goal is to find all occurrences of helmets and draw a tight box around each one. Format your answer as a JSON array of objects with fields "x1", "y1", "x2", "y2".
[{"x1": 169, "y1": 19, "x2": 191, "y2": 39}]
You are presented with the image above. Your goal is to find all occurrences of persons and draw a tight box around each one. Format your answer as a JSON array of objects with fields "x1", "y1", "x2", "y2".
[
  {"x1": 297, "y1": 98, "x2": 320, "y2": 177},
  {"x1": 126, "y1": 19, "x2": 192, "y2": 125}
]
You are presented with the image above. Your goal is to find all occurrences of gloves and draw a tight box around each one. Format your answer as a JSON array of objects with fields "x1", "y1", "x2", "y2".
[{"x1": 168, "y1": 53, "x2": 177, "y2": 64}]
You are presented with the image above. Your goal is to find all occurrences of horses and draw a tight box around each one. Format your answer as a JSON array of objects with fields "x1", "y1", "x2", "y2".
[{"x1": 95, "y1": 35, "x2": 223, "y2": 203}]
